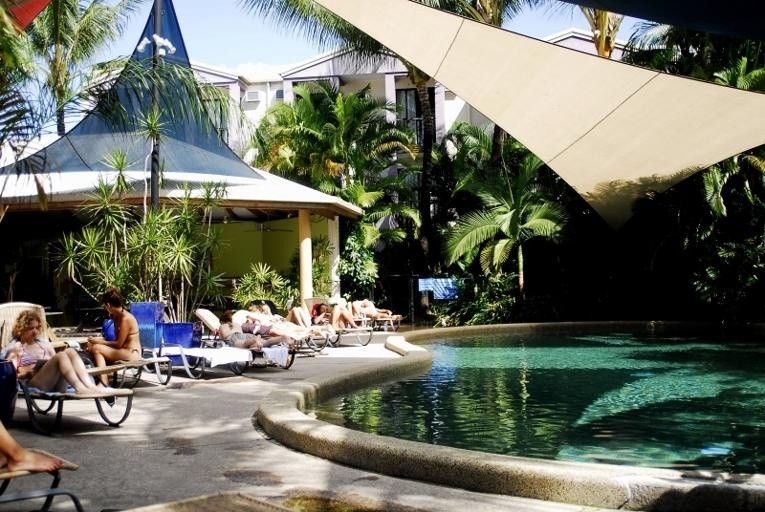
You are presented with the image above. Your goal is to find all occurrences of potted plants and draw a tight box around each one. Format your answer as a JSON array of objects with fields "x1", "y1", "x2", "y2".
[
  {"x1": 157, "y1": 179, "x2": 230, "y2": 366},
  {"x1": 43, "y1": 98, "x2": 165, "y2": 349}
]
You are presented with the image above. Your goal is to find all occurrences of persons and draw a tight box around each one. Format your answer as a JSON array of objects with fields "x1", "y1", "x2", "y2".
[
  {"x1": 5, "y1": 309, "x2": 114, "y2": 396},
  {"x1": 84, "y1": 285, "x2": 143, "y2": 387},
  {"x1": 219, "y1": 296, "x2": 403, "y2": 350},
  {"x1": 0, "y1": 419, "x2": 65, "y2": 473}
]
